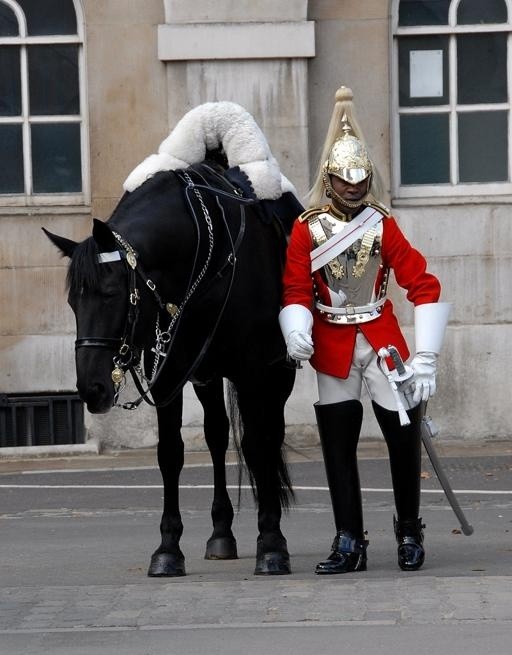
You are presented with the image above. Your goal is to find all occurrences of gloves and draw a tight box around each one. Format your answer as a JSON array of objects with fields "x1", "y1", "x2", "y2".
[
  {"x1": 278, "y1": 304, "x2": 315, "y2": 361},
  {"x1": 398, "y1": 303, "x2": 452, "y2": 402}
]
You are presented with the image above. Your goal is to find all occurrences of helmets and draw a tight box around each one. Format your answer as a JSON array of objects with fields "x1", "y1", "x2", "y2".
[{"x1": 303, "y1": 85, "x2": 385, "y2": 207}]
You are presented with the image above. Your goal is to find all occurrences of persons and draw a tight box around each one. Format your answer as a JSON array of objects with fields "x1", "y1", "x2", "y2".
[{"x1": 277, "y1": 173, "x2": 445, "y2": 576}]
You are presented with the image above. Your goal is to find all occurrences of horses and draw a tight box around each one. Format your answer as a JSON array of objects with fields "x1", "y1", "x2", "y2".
[{"x1": 40, "y1": 158, "x2": 301, "y2": 577}]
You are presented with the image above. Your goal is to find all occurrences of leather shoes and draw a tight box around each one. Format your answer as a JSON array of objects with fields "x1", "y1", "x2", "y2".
[
  {"x1": 393, "y1": 513, "x2": 426, "y2": 571},
  {"x1": 315, "y1": 530, "x2": 369, "y2": 574}
]
